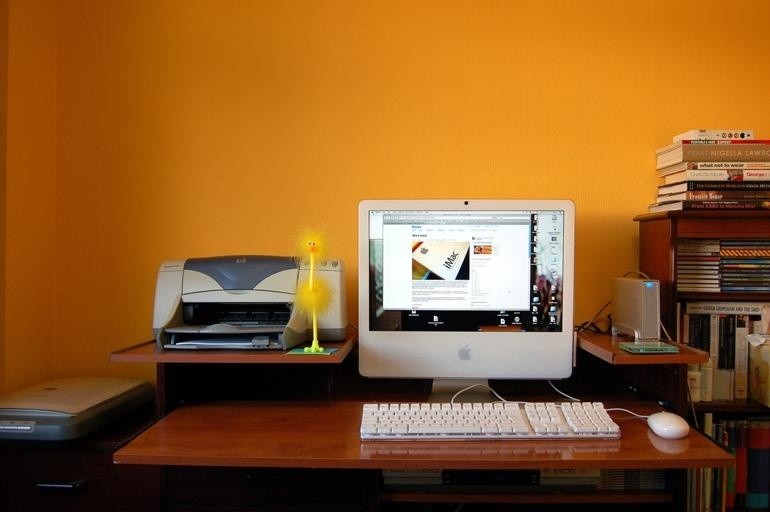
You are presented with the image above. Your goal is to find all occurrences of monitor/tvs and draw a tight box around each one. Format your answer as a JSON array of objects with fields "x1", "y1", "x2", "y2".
[{"x1": 358, "y1": 199, "x2": 575, "y2": 402}]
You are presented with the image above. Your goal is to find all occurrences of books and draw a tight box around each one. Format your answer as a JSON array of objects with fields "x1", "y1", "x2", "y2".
[
  {"x1": 689, "y1": 411, "x2": 770, "y2": 512},
  {"x1": 664, "y1": 170, "x2": 770, "y2": 187},
  {"x1": 656, "y1": 180, "x2": 770, "y2": 197},
  {"x1": 720, "y1": 241, "x2": 770, "y2": 293},
  {"x1": 681, "y1": 312, "x2": 769, "y2": 403},
  {"x1": 382, "y1": 469, "x2": 443, "y2": 486},
  {"x1": 684, "y1": 300, "x2": 769, "y2": 317},
  {"x1": 656, "y1": 190, "x2": 770, "y2": 205},
  {"x1": 655, "y1": 139, "x2": 770, "y2": 157},
  {"x1": 675, "y1": 238, "x2": 721, "y2": 292},
  {"x1": 654, "y1": 144, "x2": 770, "y2": 171},
  {"x1": 672, "y1": 127, "x2": 753, "y2": 142},
  {"x1": 648, "y1": 200, "x2": 770, "y2": 214},
  {"x1": 658, "y1": 161, "x2": 769, "y2": 178},
  {"x1": 538, "y1": 469, "x2": 601, "y2": 486}
]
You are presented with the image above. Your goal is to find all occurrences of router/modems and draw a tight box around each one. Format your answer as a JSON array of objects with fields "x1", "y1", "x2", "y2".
[{"x1": 619, "y1": 341, "x2": 681, "y2": 356}]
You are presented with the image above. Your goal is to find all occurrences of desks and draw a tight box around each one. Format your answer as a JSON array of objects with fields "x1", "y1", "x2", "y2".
[{"x1": 109, "y1": 334, "x2": 735, "y2": 512}]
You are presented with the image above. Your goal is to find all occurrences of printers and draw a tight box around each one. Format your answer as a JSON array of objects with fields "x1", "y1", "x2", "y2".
[
  {"x1": 152, "y1": 255, "x2": 347, "y2": 350},
  {"x1": 0, "y1": 378, "x2": 162, "y2": 512}
]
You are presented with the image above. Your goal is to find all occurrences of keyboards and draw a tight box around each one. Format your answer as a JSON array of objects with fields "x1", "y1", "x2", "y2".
[{"x1": 360, "y1": 402, "x2": 620, "y2": 441}]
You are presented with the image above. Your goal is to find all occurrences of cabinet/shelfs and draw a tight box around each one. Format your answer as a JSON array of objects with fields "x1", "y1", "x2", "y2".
[{"x1": 633, "y1": 209, "x2": 770, "y2": 510}]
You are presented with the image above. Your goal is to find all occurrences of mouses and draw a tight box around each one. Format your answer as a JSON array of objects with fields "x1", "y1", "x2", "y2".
[{"x1": 647, "y1": 411, "x2": 689, "y2": 439}]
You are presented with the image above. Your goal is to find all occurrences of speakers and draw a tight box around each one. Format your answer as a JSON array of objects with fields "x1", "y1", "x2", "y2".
[{"x1": 611, "y1": 278, "x2": 659, "y2": 340}]
